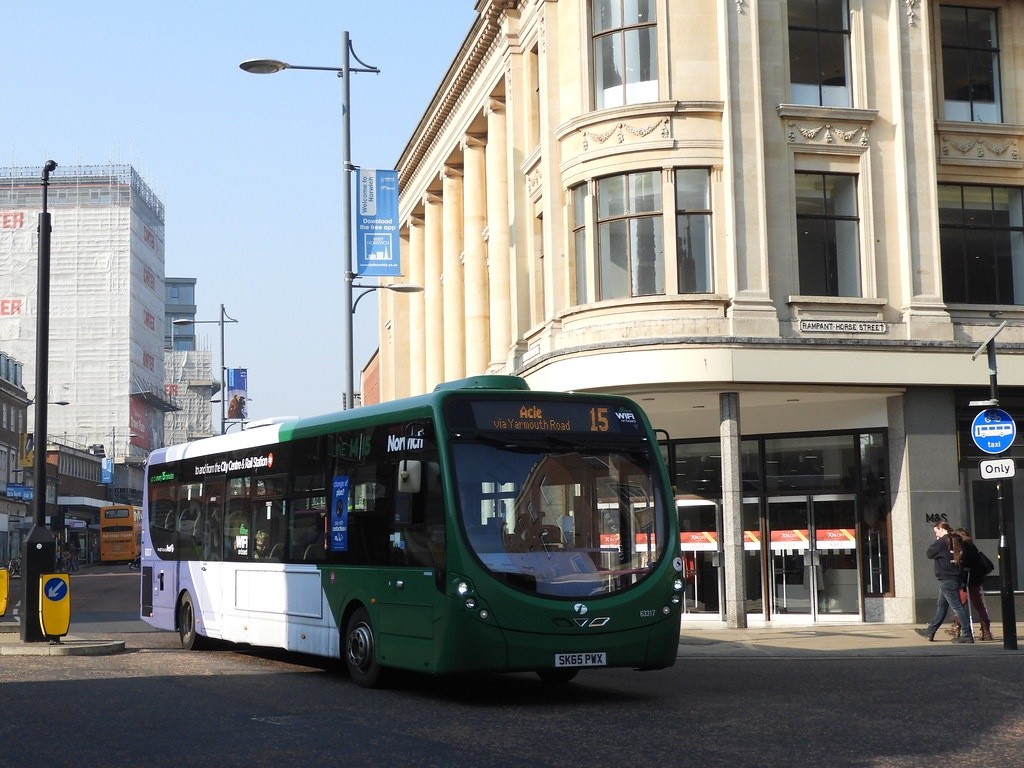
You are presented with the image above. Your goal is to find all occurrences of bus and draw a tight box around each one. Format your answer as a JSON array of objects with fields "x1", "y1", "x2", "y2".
[
  {"x1": 99, "y1": 503, "x2": 143, "y2": 561},
  {"x1": 145, "y1": 373, "x2": 687, "y2": 693}
]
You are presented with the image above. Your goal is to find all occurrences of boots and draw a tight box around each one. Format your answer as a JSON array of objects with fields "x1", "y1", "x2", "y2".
[
  {"x1": 944, "y1": 616, "x2": 961, "y2": 639},
  {"x1": 974, "y1": 618, "x2": 993, "y2": 641}
]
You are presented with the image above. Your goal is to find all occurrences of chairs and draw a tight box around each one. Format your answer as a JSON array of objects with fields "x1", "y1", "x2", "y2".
[{"x1": 151, "y1": 497, "x2": 326, "y2": 561}]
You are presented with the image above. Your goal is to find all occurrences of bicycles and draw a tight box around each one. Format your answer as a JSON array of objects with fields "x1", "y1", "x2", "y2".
[{"x1": 5, "y1": 547, "x2": 74, "y2": 580}]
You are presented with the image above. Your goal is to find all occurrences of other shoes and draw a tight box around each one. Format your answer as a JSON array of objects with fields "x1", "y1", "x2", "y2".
[
  {"x1": 914, "y1": 627, "x2": 934, "y2": 641},
  {"x1": 950, "y1": 636, "x2": 974, "y2": 643}
]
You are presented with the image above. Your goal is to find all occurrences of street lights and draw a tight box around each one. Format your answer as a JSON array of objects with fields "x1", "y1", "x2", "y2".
[
  {"x1": 173, "y1": 302, "x2": 250, "y2": 435},
  {"x1": 106, "y1": 427, "x2": 138, "y2": 506},
  {"x1": 239, "y1": 29, "x2": 425, "y2": 409}
]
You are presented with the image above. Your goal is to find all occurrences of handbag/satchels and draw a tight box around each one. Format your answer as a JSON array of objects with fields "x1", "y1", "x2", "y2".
[{"x1": 967, "y1": 551, "x2": 994, "y2": 577}]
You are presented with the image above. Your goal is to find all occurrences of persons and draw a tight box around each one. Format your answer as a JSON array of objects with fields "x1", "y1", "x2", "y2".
[
  {"x1": 914, "y1": 521, "x2": 974, "y2": 644},
  {"x1": 944, "y1": 528, "x2": 995, "y2": 641},
  {"x1": 57, "y1": 539, "x2": 79, "y2": 572},
  {"x1": 403, "y1": 522, "x2": 441, "y2": 566},
  {"x1": 227, "y1": 393, "x2": 245, "y2": 419}
]
